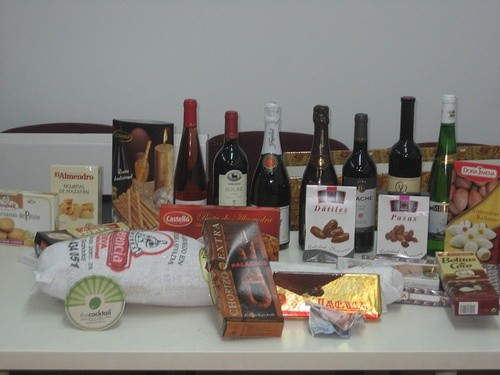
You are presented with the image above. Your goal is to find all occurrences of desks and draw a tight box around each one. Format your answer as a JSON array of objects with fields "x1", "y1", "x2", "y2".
[{"x1": 0, "y1": 201, "x2": 500, "y2": 375}]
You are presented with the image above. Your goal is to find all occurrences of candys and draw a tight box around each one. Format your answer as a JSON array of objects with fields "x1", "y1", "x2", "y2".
[
  {"x1": 385, "y1": 224, "x2": 419, "y2": 249},
  {"x1": 310, "y1": 219, "x2": 349, "y2": 243},
  {"x1": 273, "y1": 271, "x2": 382, "y2": 320}
]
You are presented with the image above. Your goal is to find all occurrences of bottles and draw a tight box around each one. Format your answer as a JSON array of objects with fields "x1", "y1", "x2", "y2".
[
  {"x1": 248, "y1": 100, "x2": 292, "y2": 250},
  {"x1": 173, "y1": 97, "x2": 210, "y2": 207},
  {"x1": 427, "y1": 94, "x2": 458, "y2": 257},
  {"x1": 340, "y1": 112, "x2": 376, "y2": 255},
  {"x1": 299, "y1": 104, "x2": 338, "y2": 253},
  {"x1": 387, "y1": 95, "x2": 420, "y2": 198},
  {"x1": 211, "y1": 110, "x2": 248, "y2": 206}
]
